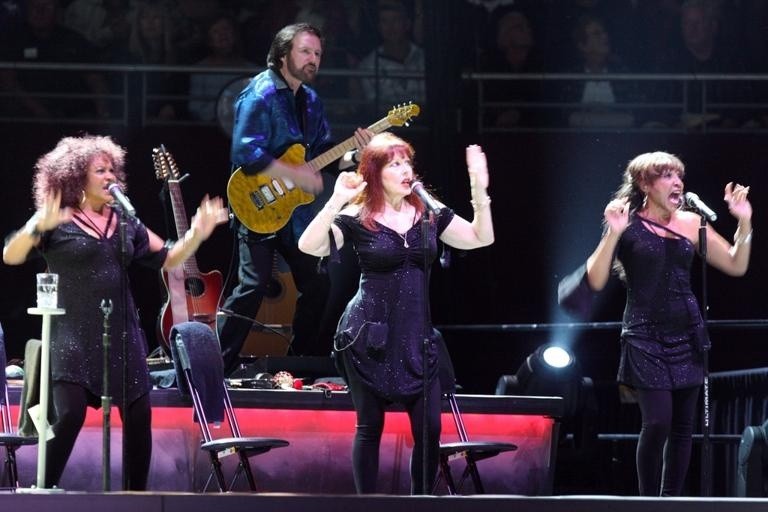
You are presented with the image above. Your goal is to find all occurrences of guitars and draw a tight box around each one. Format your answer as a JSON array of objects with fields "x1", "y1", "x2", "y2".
[
  {"x1": 240, "y1": 250, "x2": 300, "y2": 356},
  {"x1": 152, "y1": 142, "x2": 226, "y2": 352},
  {"x1": 226, "y1": 102, "x2": 420, "y2": 233}
]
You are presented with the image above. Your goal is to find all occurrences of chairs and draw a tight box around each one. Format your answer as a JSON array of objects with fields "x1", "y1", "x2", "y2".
[
  {"x1": 431, "y1": 327, "x2": 517, "y2": 495},
  {"x1": 0, "y1": 320, "x2": 40, "y2": 489},
  {"x1": 168, "y1": 319, "x2": 290, "y2": 494}
]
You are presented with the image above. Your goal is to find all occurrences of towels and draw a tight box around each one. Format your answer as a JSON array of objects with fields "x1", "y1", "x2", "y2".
[{"x1": 168, "y1": 322, "x2": 225, "y2": 424}]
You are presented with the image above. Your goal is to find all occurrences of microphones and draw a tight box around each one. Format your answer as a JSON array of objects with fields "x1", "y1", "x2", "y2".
[
  {"x1": 410, "y1": 179, "x2": 441, "y2": 216},
  {"x1": 685, "y1": 190, "x2": 718, "y2": 222},
  {"x1": 106, "y1": 180, "x2": 136, "y2": 218}
]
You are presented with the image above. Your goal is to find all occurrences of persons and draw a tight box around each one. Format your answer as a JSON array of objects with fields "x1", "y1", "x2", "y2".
[
  {"x1": 297, "y1": 131, "x2": 495, "y2": 495},
  {"x1": 3, "y1": 135, "x2": 231, "y2": 492},
  {"x1": 214, "y1": 22, "x2": 378, "y2": 380},
  {"x1": 586, "y1": 150, "x2": 753, "y2": 500},
  {"x1": 1, "y1": 0, "x2": 722, "y2": 125}
]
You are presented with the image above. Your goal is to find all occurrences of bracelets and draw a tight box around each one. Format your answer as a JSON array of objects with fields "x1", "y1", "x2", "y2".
[{"x1": 470, "y1": 196, "x2": 492, "y2": 213}]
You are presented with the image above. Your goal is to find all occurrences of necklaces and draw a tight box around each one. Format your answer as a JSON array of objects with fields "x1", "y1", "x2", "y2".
[{"x1": 382, "y1": 213, "x2": 415, "y2": 249}]
